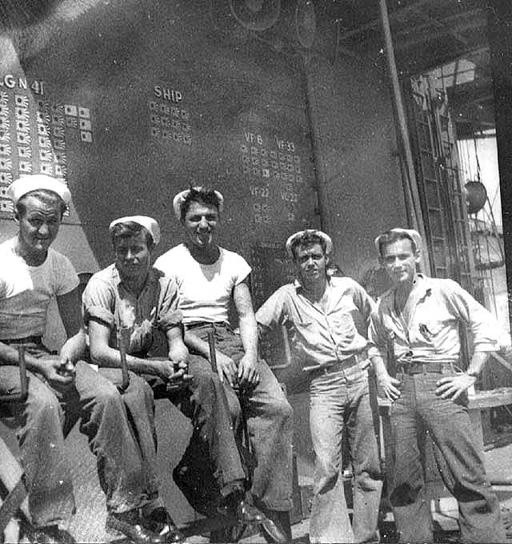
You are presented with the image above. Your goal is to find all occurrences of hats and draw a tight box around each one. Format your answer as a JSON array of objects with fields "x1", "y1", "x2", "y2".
[
  {"x1": 8, "y1": 175, "x2": 73, "y2": 204},
  {"x1": 286, "y1": 229, "x2": 333, "y2": 258},
  {"x1": 375, "y1": 228, "x2": 422, "y2": 258},
  {"x1": 173, "y1": 187, "x2": 224, "y2": 219},
  {"x1": 109, "y1": 216, "x2": 160, "y2": 246}
]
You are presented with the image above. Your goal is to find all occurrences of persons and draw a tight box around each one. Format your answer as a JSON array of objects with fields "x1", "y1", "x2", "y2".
[
  {"x1": 237, "y1": 228, "x2": 387, "y2": 543},
  {"x1": 367, "y1": 228, "x2": 511, "y2": 544},
  {"x1": 0, "y1": 175, "x2": 162, "y2": 543},
  {"x1": 153, "y1": 186, "x2": 295, "y2": 543},
  {"x1": 79, "y1": 216, "x2": 266, "y2": 544}
]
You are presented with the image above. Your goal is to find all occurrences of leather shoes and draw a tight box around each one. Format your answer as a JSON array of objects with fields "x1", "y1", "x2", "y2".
[
  {"x1": 148, "y1": 509, "x2": 185, "y2": 543},
  {"x1": 106, "y1": 511, "x2": 164, "y2": 543},
  {"x1": 259, "y1": 511, "x2": 288, "y2": 543},
  {"x1": 218, "y1": 501, "x2": 266, "y2": 522},
  {"x1": 36, "y1": 529, "x2": 73, "y2": 544}
]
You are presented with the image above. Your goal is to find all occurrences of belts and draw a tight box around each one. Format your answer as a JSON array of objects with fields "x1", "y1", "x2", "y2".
[
  {"x1": 395, "y1": 363, "x2": 463, "y2": 374},
  {"x1": 313, "y1": 351, "x2": 367, "y2": 373},
  {"x1": 1, "y1": 334, "x2": 44, "y2": 345},
  {"x1": 189, "y1": 322, "x2": 233, "y2": 331}
]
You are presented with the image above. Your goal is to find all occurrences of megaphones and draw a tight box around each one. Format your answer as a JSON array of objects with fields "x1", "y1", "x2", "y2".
[
  {"x1": 283, "y1": 3, "x2": 317, "y2": 49},
  {"x1": 229, "y1": 0, "x2": 281, "y2": 30}
]
